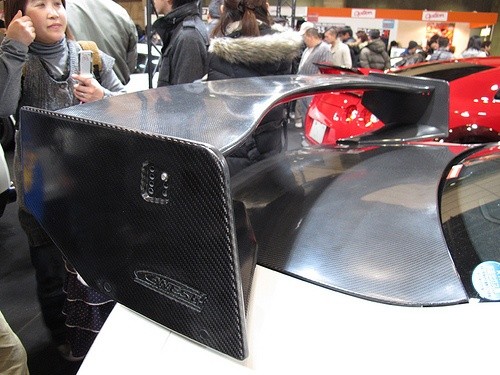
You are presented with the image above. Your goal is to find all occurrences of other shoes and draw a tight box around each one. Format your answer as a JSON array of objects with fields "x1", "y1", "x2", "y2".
[
  {"x1": 56, "y1": 344, "x2": 85, "y2": 362},
  {"x1": 289, "y1": 112, "x2": 295, "y2": 119},
  {"x1": 295, "y1": 122, "x2": 302, "y2": 127}
]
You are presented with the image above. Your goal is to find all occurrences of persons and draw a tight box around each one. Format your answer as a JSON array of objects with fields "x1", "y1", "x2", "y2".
[
  {"x1": 66, "y1": 0, "x2": 163, "y2": 84},
  {"x1": 274, "y1": 18, "x2": 491, "y2": 75},
  {"x1": 207, "y1": 0, "x2": 302, "y2": 80},
  {"x1": 0, "y1": 310, "x2": 30, "y2": 375},
  {"x1": 152, "y1": 0, "x2": 209, "y2": 88},
  {"x1": 0, "y1": 0, "x2": 128, "y2": 360}
]
